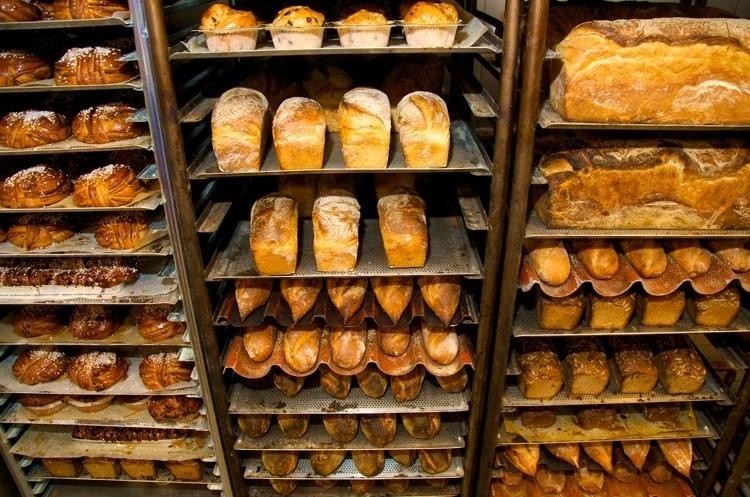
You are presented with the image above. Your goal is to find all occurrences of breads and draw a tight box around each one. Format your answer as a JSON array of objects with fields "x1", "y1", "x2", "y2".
[
  {"x1": 491, "y1": 1, "x2": 747, "y2": 497},
  {"x1": 0, "y1": 1, "x2": 202, "y2": 497},
  {"x1": 198, "y1": 1, "x2": 469, "y2": 496}
]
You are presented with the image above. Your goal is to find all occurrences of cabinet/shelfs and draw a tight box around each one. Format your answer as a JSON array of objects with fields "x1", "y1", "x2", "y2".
[
  {"x1": 138, "y1": 0, "x2": 525, "y2": 496},
  {"x1": 459, "y1": 0, "x2": 750, "y2": 496},
  {"x1": 0, "y1": 0, "x2": 239, "y2": 497}
]
